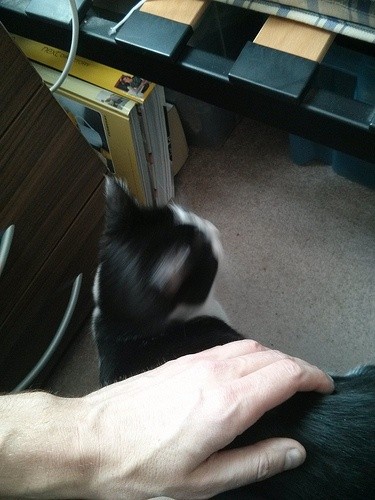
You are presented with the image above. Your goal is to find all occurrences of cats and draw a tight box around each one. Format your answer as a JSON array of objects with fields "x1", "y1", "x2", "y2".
[{"x1": 90, "y1": 172, "x2": 375, "y2": 500}]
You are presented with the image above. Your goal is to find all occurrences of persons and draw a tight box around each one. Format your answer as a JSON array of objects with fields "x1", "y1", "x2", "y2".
[
  {"x1": 120, "y1": 74, "x2": 149, "y2": 94},
  {"x1": 0, "y1": 337, "x2": 334, "y2": 500}
]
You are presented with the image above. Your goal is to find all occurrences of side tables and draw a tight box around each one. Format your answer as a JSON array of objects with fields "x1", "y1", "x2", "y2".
[{"x1": 0, "y1": 19, "x2": 129, "y2": 396}]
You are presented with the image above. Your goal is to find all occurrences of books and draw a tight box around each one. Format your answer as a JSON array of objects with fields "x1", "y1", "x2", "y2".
[
  {"x1": 8, "y1": 33, "x2": 177, "y2": 208},
  {"x1": 30, "y1": 61, "x2": 153, "y2": 210}
]
